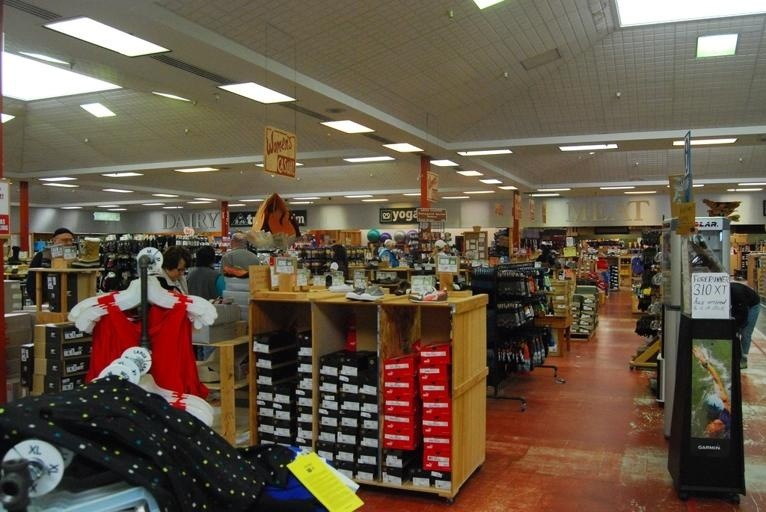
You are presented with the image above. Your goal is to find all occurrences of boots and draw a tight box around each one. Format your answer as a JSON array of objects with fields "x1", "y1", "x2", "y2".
[
  {"x1": 8, "y1": 246, "x2": 20, "y2": 260},
  {"x1": 71, "y1": 237, "x2": 101, "y2": 268}
]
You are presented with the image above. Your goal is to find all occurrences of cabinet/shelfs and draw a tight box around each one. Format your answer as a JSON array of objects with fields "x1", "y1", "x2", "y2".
[
  {"x1": 191, "y1": 335, "x2": 249, "y2": 450},
  {"x1": 617, "y1": 255, "x2": 632, "y2": 290},
  {"x1": 248, "y1": 293, "x2": 490, "y2": 501}
]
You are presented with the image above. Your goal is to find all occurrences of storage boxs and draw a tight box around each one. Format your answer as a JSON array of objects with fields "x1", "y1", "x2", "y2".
[
  {"x1": 318, "y1": 349, "x2": 378, "y2": 483},
  {"x1": 251, "y1": 328, "x2": 313, "y2": 456},
  {"x1": 381, "y1": 341, "x2": 451, "y2": 492},
  {"x1": 196, "y1": 303, "x2": 239, "y2": 341}
]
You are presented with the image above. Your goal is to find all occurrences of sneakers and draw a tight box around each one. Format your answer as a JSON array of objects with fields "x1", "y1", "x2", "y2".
[
  {"x1": 346, "y1": 286, "x2": 385, "y2": 300},
  {"x1": 409, "y1": 288, "x2": 448, "y2": 304}
]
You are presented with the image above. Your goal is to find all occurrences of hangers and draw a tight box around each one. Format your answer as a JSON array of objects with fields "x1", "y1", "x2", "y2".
[{"x1": 66, "y1": 247, "x2": 220, "y2": 429}]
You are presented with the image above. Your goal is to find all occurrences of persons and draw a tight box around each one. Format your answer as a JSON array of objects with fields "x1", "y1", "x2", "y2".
[
  {"x1": 435, "y1": 239, "x2": 447, "y2": 255},
  {"x1": 26, "y1": 228, "x2": 74, "y2": 305},
  {"x1": 730, "y1": 282, "x2": 761, "y2": 368},
  {"x1": 321, "y1": 245, "x2": 349, "y2": 280},
  {"x1": 187, "y1": 246, "x2": 226, "y2": 302},
  {"x1": 221, "y1": 233, "x2": 260, "y2": 278},
  {"x1": 162, "y1": 246, "x2": 191, "y2": 297},
  {"x1": 378, "y1": 239, "x2": 400, "y2": 268}
]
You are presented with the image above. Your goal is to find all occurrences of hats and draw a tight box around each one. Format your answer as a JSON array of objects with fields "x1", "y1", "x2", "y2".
[
  {"x1": 53, "y1": 227, "x2": 73, "y2": 237},
  {"x1": 435, "y1": 240, "x2": 448, "y2": 249}
]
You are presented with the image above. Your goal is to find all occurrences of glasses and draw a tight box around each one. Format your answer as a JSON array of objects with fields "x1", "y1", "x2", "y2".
[{"x1": 176, "y1": 268, "x2": 186, "y2": 272}]
